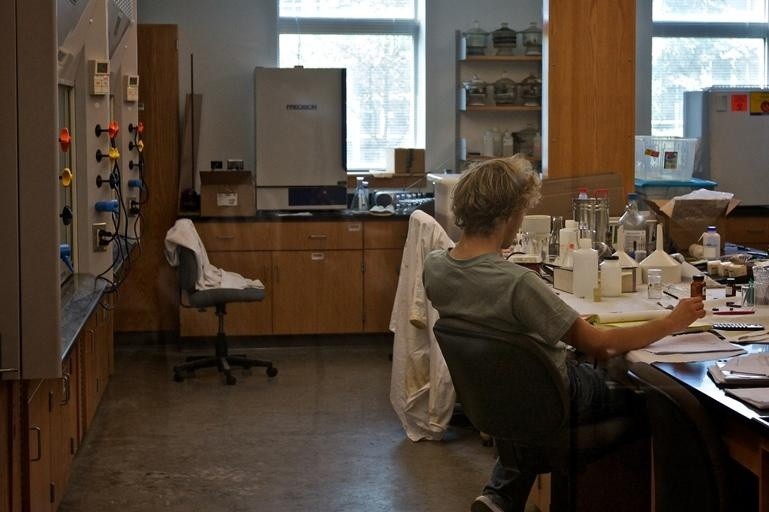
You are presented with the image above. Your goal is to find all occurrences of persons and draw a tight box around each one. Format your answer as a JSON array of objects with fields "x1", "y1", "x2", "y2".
[{"x1": 422, "y1": 153, "x2": 706, "y2": 512}]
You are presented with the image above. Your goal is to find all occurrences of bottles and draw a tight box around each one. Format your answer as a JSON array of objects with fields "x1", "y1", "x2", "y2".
[
  {"x1": 350, "y1": 176, "x2": 370, "y2": 212},
  {"x1": 480, "y1": 126, "x2": 542, "y2": 159},
  {"x1": 464, "y1": 19, "x2": 543, "y2": 56},
  {"x1": 647, "y1": 268, "x2": 663, "y2": 299},
  {"x1": 724, "y1": 276, "x2": 737, "y2": 298},
  {"x1": 616, "y1": 191, "x2": 649, "y2": 262},
  {"x1": 690, "y1": 274, "x2": 707, "y2": 301},
  {"x1": 557, "y1": 220, "x2": 622, "y2": 303},
  {"x1": 702, "y1": 225, "x2": 722, "y2": 261},
  {"x1": 463, "y1": 71, "x2": 542, "y2": 108}
]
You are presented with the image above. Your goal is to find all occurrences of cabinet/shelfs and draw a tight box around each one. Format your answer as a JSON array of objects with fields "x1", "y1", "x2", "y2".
[
  {"x1": 364, "y1": 220, "x2": 409, "y2": 337},
  {"x1": 179, "y1": 221, "x2": 364, "y2": 337},
  {"x1": 455, "y1": 29, "x2": 542, "y2": 173},
  {"x1": 0, "y1": 283, "x2": 114, "y2": 512}
]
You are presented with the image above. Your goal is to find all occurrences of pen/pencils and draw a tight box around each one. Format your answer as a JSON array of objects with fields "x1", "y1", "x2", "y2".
[
  {"x1": 713, "y1": 310, "x2": 755, "y2": 315},
  {"x1": 663, "y1": 291, "x2": 678, "y2": 299},
  {"x1": 726, "y1": 304, "x2": 742, "y2": 308}
]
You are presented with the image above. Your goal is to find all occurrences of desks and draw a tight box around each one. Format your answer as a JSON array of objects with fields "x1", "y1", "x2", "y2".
[
  {"x1": 636, "y1": 176, "x2": 719, "y2": 212},
  {"x1": 540, "y1": 274, "x2": 769, "y2": 512}
]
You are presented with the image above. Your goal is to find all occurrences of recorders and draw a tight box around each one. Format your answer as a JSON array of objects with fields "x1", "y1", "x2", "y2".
[{"x1": 372, "y1": 187, "x2": 436, "y2": 210}]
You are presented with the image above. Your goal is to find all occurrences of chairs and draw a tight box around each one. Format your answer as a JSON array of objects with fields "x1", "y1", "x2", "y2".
[
  {"x1": 390, "y1": 210, "x2": 465, "y2": 441},
  {"x1": 165, "y1": 219, "x2": 278, "y2": 385},
  {"x1": 434, "y1": 319, "x2": 669, "y2": 512}
]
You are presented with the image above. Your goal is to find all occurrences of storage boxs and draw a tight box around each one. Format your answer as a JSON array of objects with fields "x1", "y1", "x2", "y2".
[
  {"x1": 644, "y1": 197, "x2": 742, "y2": 252},
  {"x1": 634, "y1": 136, "x2": 698, "y2": 181},
  {"x1": 199, "y1": 170, "x2": 256, "y2": 218}
]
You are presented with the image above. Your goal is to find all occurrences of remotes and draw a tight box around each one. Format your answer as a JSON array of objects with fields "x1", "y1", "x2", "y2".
[{"x1": 712, "y1": 322, "x2": 764, "y2": 331}]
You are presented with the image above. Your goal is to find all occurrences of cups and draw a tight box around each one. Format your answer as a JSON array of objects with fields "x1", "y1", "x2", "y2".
[
  {"x1": 741, "y1": 285, "x2": 756, "y2": 307},
  {"x1": 524, "y1": 230, "x2": 537, "y2": 255}
]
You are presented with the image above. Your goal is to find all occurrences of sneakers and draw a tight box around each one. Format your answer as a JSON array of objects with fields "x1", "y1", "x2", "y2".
[{"x1": 471, "y1": 496, "x2": 503, "y2": 512}]
